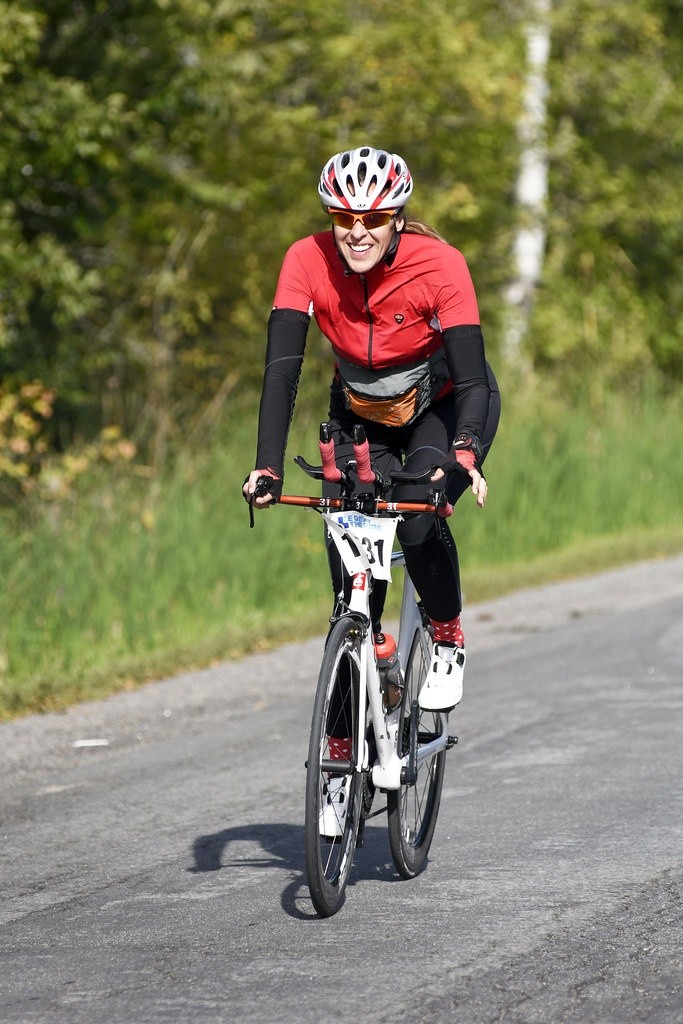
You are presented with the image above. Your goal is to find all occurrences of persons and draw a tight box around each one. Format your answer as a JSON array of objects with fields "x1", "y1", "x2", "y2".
[{"x1": 243, "y1": 149, "x2": 500, "y2": 845}]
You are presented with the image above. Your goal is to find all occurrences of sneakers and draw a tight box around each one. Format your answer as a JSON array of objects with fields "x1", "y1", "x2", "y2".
[
  {"x1": 319, "y1": 773, "x2": 353, "y2": 841},
  {"x1": 417, "y1": 640, "x2": 466, "y2": 712}
]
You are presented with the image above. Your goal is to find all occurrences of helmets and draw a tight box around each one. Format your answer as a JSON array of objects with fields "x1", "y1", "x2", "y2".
[{"x1": 318, "y1": 146, "x2": 414, "y2": 212}]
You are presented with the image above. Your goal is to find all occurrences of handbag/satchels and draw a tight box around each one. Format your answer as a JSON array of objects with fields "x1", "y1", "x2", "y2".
[{"x1": 336, "y1": 349, "x2": 447, "y2": 428}]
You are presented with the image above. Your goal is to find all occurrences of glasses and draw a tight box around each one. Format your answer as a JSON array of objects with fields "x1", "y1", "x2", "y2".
[{"x1": 328, "y1": 209, "x2": 396, "y2": 230}]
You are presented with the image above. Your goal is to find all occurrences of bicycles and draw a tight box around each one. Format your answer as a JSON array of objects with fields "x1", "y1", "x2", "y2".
[{"x1": 245, "y1": 423, "x2": 471, "y2": 919}]
